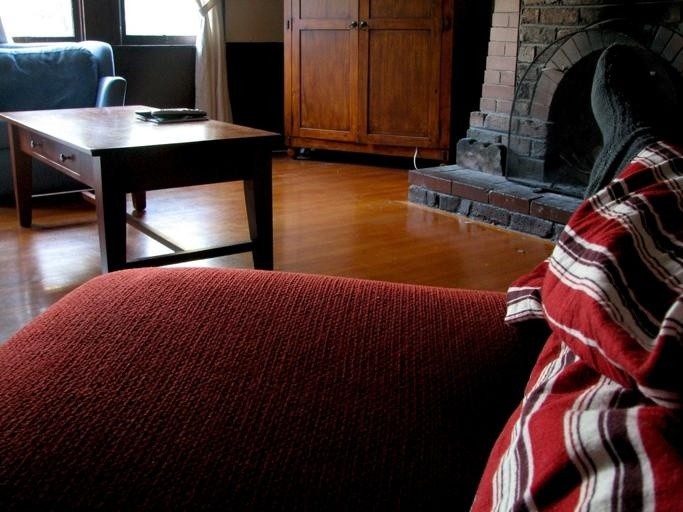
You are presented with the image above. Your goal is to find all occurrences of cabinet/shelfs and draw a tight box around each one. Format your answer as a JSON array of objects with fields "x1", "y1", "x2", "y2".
[{"x1": 282, "y1": 0, "x2": 457, "y2": 162}]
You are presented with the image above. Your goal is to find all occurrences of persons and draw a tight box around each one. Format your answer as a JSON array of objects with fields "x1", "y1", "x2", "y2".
[{"x1": 465, "y1": 39, "x2": 681, "y2": 511}]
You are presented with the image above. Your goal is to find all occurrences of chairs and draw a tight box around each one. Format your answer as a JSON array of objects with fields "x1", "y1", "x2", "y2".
[{"x1": 0, "y1": 39, "x2": 128, "y2": 201}]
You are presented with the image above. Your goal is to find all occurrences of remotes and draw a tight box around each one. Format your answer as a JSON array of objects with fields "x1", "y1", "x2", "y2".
[{"x1": 151, "y1": 108, "x2": 207, "y2": 118}]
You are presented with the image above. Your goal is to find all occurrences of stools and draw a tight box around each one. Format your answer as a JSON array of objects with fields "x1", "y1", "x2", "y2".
[{"x1": 0, "y1": 265, "x2": 551, "y2": 512}]
